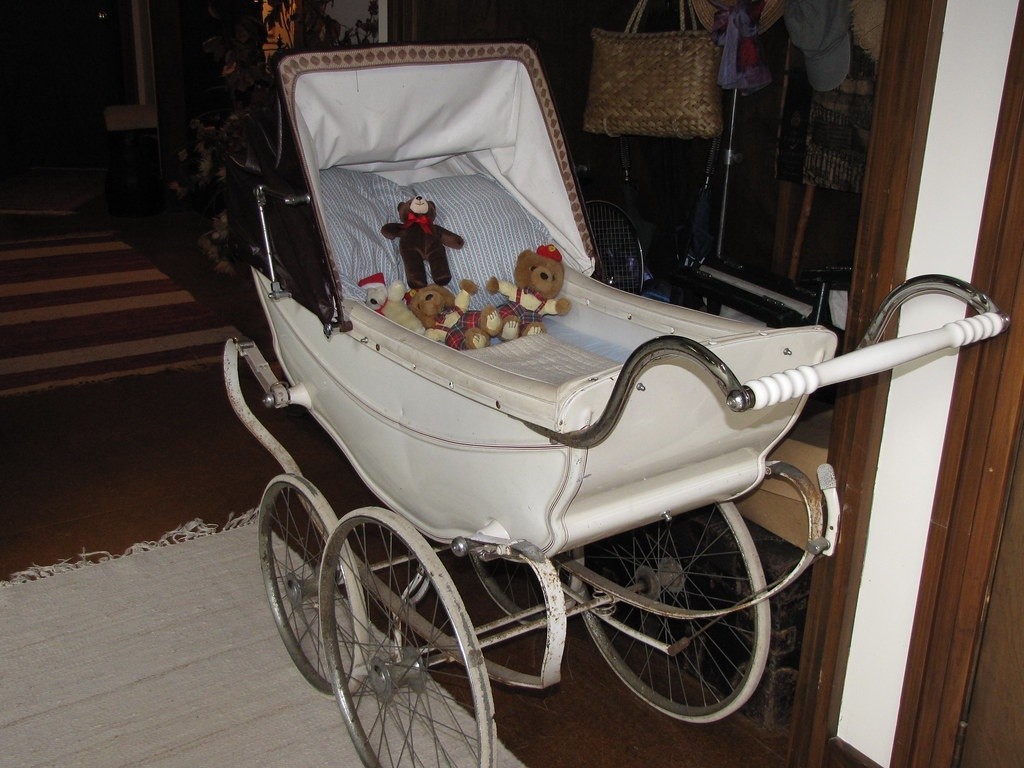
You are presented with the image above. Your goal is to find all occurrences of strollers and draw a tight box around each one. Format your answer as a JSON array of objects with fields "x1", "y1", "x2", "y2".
[{"x1": 224, "y1": 41, "x2": 1010, "y2": 768}]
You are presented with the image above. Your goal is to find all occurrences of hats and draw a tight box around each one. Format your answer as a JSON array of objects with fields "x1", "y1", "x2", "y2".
[
  {"x1": 782, "y1": 0, "x2": 851, "y2": 92},
  {"x1": 692, "y1": 0, "x2": 786, "y2": 40}
]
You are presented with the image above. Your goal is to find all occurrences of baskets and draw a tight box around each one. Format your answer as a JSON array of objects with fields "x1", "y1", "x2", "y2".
[{"x1": 583, "y1": 0, "x2": 724, "y2": 138}]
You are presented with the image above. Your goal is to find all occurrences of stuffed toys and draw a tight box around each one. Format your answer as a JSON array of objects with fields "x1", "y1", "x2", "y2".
[
  {"x1": 355, "y1": 271, "x2": 426, "y2": 338},
  {"x1": 380, "y1": 195, "x2": 465, "y2": 289},
  {"x1": 485, "y1": 243, "x2": 571, "y2": 343},
  {"x1": 849, "y1": 1, "x2": 887, "y2": 56},
  {"x1": 404, "y1": 279, "x2": 503, "y2": 348}
]
